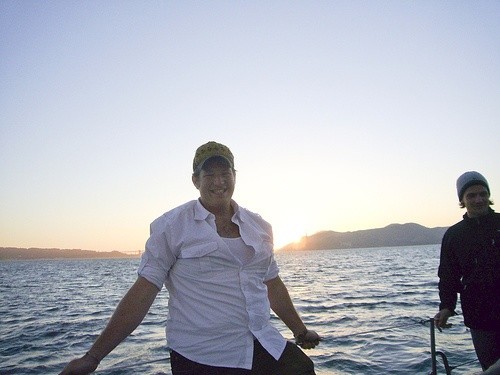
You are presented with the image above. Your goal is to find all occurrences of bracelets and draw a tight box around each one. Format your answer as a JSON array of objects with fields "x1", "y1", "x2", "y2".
[
  {"x1": 86, "y1": 351, "x2": 101, "y2": 366},
  {"x1": 294, "y1": 328, "x2": 308, "y2": 339}
]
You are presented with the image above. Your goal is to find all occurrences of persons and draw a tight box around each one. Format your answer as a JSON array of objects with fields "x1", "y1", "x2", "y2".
[
  {"x1": 58, "y1": 141, "x2": 324, "y2": 375},
  {"x1": 431, "y1": 170, "x2": 500, "y2": 375}
]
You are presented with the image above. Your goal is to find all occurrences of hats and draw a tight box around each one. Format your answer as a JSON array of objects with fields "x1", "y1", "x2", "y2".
[
  {"x1": 192, "y1": 140, "x2": 235, "y2": 173},
  {"x1": 456, "y1": 171, "x2": 490, "y2": 202}
]
